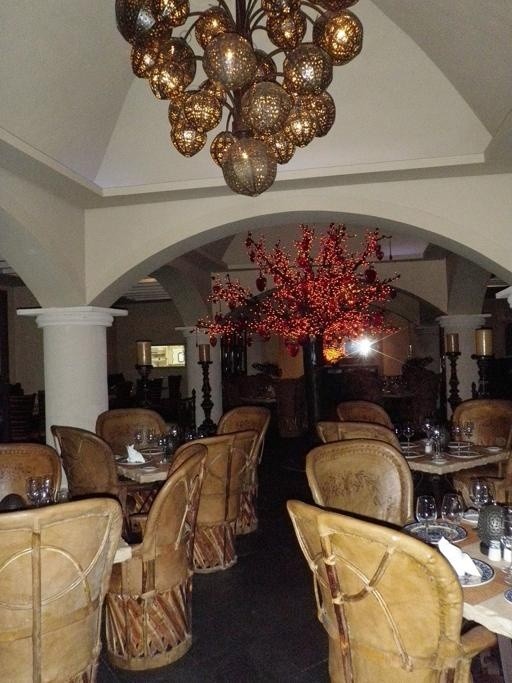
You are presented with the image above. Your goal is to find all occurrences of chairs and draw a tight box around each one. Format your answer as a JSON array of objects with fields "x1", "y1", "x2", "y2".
[
  {"x1": 316, "y1": 420, "x2": 402, "y2": 453},
  {"x1": 217, "y1": 406, "x2": 271, "y2": 535},
  {"x1": 96, "y1": 408, "x2": 169, "y2": 515},
  {"x1": 275, "y1": 378, "x2": 306, "y2": 438},
  {"x1": 50, "y1": 425, "x2": 157, "y2": 543},
  {"x1": 0, "y1": 381, "x2": 45, "y2": 442},
  {"x1": 103, "y1": 442, "x2": 208, "y2": 670},
  {"x1": 234, "y1": 375, "x2": 268, "y2": 404},
  {"x1": 173, "y1": 388, "x2": 197, "y2": 440},
  {"x1": 0, "y1": 497, "x2": 122, "y2": 683},
  {"x1": 145, "y1": 379, "x2": 163, "y2": 408},
  {"x1": 337, "y1": 399, "x2": 392, "y2": 431},
  {"x1": 287, "y1": 498, "x2": 496, "y2": 683},
  {"x1": 161, "y1": 374, "x2": 181, "y2": 405},
  {"x1": 1, "y1": 442, "x2": 62, "y2": 508},
  {"x1": 452, "y1": 399, "x2": 512, "y2": 504},
  {"x1": 306, "y1": 439, "x2": 416, "y2": 531},
  {"x1": 108, "y1": 372, "x2": 135, "y2": 408},
  {"x1": 131, "y1": 428, "x2": 259, "y2": 574}
]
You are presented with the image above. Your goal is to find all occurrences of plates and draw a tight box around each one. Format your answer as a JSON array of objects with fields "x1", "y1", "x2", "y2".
[
  {"x1": 405, "y1": 455, "x2": 425, "y2": 459},
  {"x1": 449, "y1": 451, "x2": 482, "y2": 459},
  {"x1": 460, "y1": 557, "x2": 495, "y2": 588},
  {"x1": 116, "y1": 457, "x2": 150, "y2": 466},
  {"x1": 400, "y1": 445, "x2": 419, "y2": 448},
  {"x1": 402, "y1": 521, "x2": 468, "y2": 545},
  {"x1": 448, "y1": 442, "x2": 471, "y2": 447},
  {"x1": 461, "y1": 511, "x2": 480, "y2": 524},
  {"x1": 504, "y1": 589, "x2": 512, "y2": 604},
  {"x1": 141, "y1": 448, "x2": 164, "y2": 455},
  {"x1": 487, "y1": 447, "x2": 499, "y2": 451}
]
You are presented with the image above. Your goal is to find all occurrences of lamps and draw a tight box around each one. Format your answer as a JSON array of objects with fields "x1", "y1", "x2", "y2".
[
  {"x1": 136, "y1": 340, "x2": 152, "y2": 408},
  {"x1": 199, "y1": 343, "x2": 217, "y2": 433},
  {"x1": 471, "y1": 328, "x2": 497, "y2": 399},
  {"x1": 115, "y1": 0, "x2": 363, "y2": 197},
  {"x1": 445, "y1": 333, "x2": 462, "y2": 410}
]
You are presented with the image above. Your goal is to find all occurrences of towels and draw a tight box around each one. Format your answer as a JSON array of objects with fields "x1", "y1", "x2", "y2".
[
  {"x1": 436, "y1": 534, "x2": 485, "y2": 580},
  {"x1": 122, "y1": 441, "x2": 147, "y2": 464}
]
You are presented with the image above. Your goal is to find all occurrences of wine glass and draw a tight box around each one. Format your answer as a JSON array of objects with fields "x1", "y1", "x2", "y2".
[
  {"x1": 450, "y1": 424, "x2": 464, "y2": 451},
  {"x1": 26, "y1": 476, "x2": 51, "y2": 508},
  {"x1": 422, "y1": 423, "x2": 433, "y2": 446},
  {"x1": 145, "y1": 432, "x2": 154, "y2": 450},
  {"x1": 171, "y1": 429, "x2": 178, "y2": 448},
  {"x1": 501, "y1": 520, "x2": 512, "y2": 573},
  {"x1": 463, "y1": 421, "x2": 474, "y2": 449},
  {"x1": 403, "y1": 425, "x2": 415, "y2": 452},
  {"x1": 394, "y1": 424, "x2": 402, "y2": 439},
  {"x1": 431, "y1": 424, "x2": 446, "y2": 464},
  {"x1": 442, "y1": 494, "x2": 464, "y2": 548},
  {"x1": 198, "y1": 431, "x2": 207, "y2": 439},
  {"x1": 469, "y1": 482, "x2": 488, "y2": 517},
  {"x1": 416, "y1": 495, "x2": 435, "y2": 545},
  {"x1": 134, "y1": 428, "x2": 144, "y2": 451}
]
